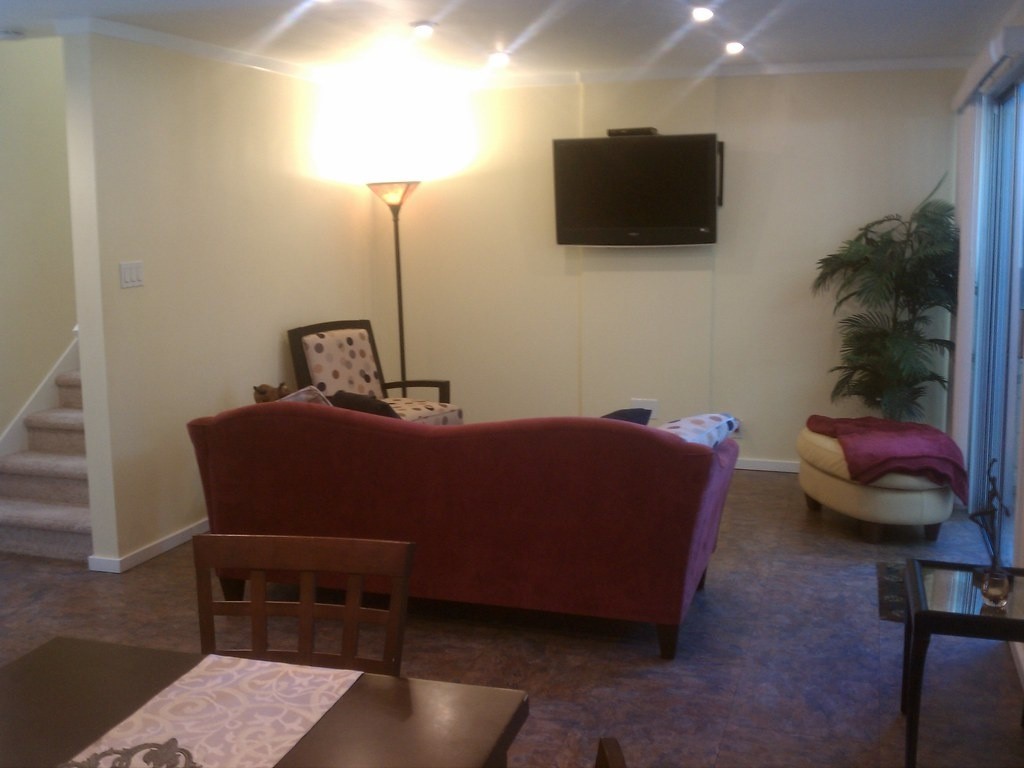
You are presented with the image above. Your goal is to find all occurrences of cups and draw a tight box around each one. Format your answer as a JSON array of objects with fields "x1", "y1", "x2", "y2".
[{"x1": 980, "y1": 572, "x2": 1010, "y2": 608}]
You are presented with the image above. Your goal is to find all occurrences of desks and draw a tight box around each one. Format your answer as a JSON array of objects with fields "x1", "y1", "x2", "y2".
[
  {"x1": 0, "y1": 633, "x2": 533, "y2": 768},
  {"x1": 899, "y1": 555, "x2": 1024, "y2": 768}
]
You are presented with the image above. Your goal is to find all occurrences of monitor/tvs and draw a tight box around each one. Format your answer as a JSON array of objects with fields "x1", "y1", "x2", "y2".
[{"x1": 553, "y1": 133, "x2": 718, "y2": 246}]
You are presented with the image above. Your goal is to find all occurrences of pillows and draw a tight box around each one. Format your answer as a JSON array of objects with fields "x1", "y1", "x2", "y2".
[
  {"x1": 330, "y1": 390, "x2": 401, "y2": 418},
  {"x1": 656, "y1": 412, "x2": 744, "y2": 452},
  {"x1": 601, "y1": 408, "x2": 653, "y2": 425},
  {"x1": 275, "y1": 386, "x2": 330, "y2": 405}
]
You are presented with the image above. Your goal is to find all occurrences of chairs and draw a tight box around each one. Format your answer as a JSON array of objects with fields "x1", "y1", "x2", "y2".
[
  {"x1": 191, "y1": 531, "x2": 415, "y2": 679},
  {"x1": 286, "y1": 319, "x2": 465, "y2": 426}
]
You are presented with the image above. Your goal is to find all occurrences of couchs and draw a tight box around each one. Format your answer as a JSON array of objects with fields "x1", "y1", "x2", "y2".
[{"x1": 184, "y1": 399, "x2": 744, "y2": 662}]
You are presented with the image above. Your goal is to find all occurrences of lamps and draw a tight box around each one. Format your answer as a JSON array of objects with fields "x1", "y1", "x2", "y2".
[{"x1": 365, "y1": 180, "x2": 421, "y2": 398}]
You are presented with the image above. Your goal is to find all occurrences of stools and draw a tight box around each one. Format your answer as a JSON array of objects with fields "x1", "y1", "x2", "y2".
[{"x1": 795, "y1": 414, "x2": 954, "y2": 544}]
